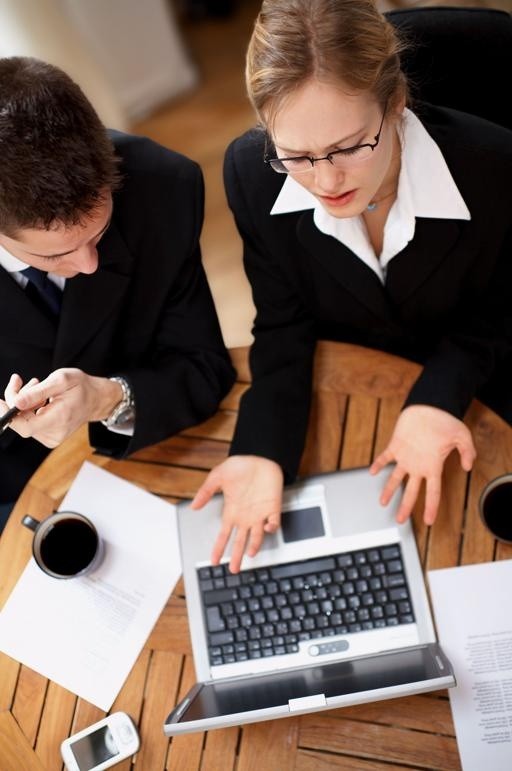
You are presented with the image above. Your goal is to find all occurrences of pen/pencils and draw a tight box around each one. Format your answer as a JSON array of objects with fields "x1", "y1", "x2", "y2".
[{"x1": 0, "y1": 408, "x2": 18, "y2": 426}]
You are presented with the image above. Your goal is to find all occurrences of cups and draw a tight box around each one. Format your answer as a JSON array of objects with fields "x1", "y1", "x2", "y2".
[
  {"x1": 20, "y1": 510, "x2": 105, "y2": 580},
  {"x1": 479, "y1": 473, "x2": 512, "y2": 548}
]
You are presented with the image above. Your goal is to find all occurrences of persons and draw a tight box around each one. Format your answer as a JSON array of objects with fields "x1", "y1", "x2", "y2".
[
  {"x1": 191, "y1": 0, "x2": 512, "y2": 576},
  {"x1": 0, "y1": 50, "x2": 242, "y2": 538}
]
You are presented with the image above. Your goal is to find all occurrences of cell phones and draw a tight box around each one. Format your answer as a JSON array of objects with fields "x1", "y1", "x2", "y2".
[{"x1": 60, "y1": 711, "x2": 140, "y2": 771}]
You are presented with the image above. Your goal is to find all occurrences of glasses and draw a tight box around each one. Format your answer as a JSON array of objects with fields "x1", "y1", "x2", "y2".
[{"x1": 263, "y1": 99, "x2": 389, "y2": 175}]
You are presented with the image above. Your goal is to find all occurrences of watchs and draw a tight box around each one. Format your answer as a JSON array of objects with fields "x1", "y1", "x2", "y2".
[{"x1": 101, "y1": 372, "x2": 137, "y2": 434}]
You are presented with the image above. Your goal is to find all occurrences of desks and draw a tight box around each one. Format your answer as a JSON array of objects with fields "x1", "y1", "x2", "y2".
[{"x1": 1, "y1": 340, "x2": 511, "y2": 768}]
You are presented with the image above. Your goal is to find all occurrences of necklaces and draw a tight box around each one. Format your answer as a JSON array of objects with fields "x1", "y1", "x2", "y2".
[{"x1": 365, "y1": 187, "x2": 396, "y2": 213}]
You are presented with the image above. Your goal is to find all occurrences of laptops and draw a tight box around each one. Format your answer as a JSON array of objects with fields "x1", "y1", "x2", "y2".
[{"x1": 156, "y1": 465, "x2": 465, "y2": 737}]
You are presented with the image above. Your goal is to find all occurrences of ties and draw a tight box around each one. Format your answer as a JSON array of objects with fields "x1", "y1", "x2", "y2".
[{"x1": 19, "y1": 266, "x2": 62, "y2": 322}]
